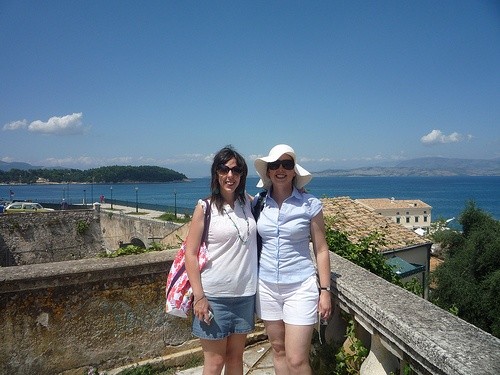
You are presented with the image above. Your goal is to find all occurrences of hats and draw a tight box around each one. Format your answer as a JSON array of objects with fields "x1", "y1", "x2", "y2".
[{"x1": 254, "y1": 144, "x2": 313, "y2": 190}]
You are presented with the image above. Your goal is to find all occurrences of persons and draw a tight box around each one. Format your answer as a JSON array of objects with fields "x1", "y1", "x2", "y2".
[
  {"x1": 184, "y1": 147, "x2": 259, "y2": 375},
  {"x1": 256, "y1": 144, "x2": 332, "y2": 375}
]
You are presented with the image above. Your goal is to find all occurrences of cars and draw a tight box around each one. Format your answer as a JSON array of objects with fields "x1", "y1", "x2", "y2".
[{"x1": 6, "y1": 204, "x2": 51, "y2": 213}]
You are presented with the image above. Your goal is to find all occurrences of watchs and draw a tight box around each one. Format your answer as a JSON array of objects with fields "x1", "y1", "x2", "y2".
[{"x1": 320, "y1": 286, "x2": 331, "y2": 292}]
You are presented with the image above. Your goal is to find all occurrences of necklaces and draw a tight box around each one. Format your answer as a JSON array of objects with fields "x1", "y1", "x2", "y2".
[{"x1": 222, "y1": 196, "x2": 250, "y2": 244}]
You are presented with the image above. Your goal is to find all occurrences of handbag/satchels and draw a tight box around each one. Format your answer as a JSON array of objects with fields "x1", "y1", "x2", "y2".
[{"x1": 166, "y1": 199, "x2": 210, "y2": 319}]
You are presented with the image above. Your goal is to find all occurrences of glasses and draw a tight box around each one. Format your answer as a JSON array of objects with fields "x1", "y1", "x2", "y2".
[
  {"x1": 216, "y1": 164, "x2": 245, "y2": 176},
  {"x1": 268, "y1": 160, "x2": 294, "y2": 170}
]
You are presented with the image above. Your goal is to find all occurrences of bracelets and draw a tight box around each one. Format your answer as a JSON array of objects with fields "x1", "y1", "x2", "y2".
[{"x1": 194, "y1": 295, "x2": 206, "y2": 306}]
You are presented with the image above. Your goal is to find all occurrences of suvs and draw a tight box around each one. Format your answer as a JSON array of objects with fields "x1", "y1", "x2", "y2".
[{"x1": 5, "y1": 202, "x2": 54, "y2": 212}]
